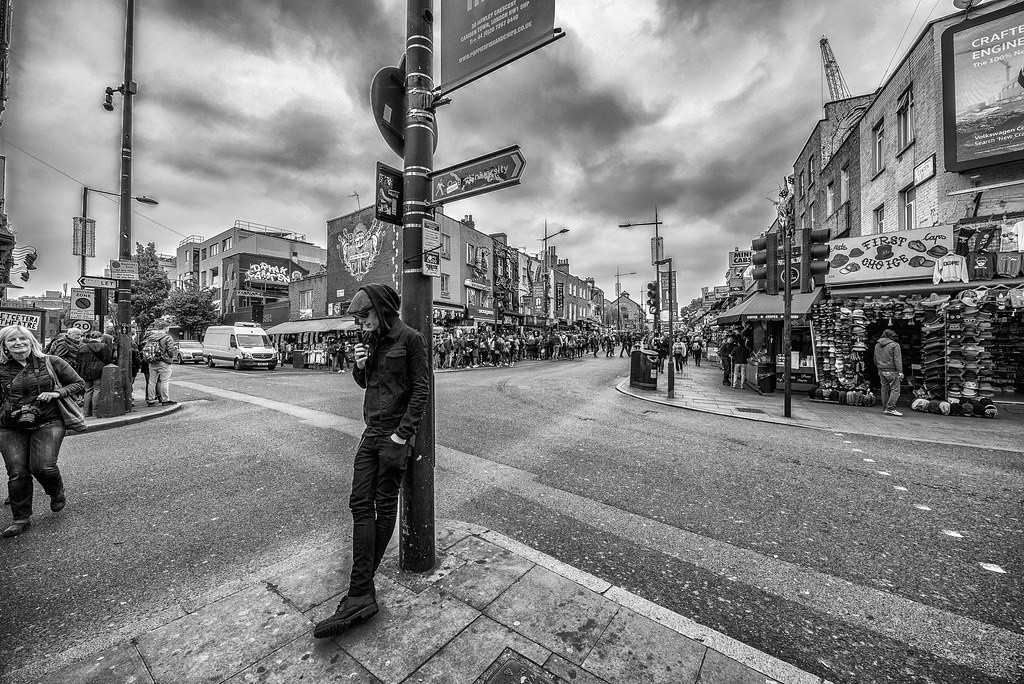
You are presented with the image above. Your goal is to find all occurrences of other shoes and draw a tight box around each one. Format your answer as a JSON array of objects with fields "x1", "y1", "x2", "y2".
[
  {"x1": 723, "y1": 382, "x2": 731, "y2": 386},
  {"x1": 51, "y1": 486, "x2": 66, "y2": 512},
  {"x1": 162, "y1": 400, "x2": 177, "y2": 407},
  {"x1": 434, "y1": 353, "x2": 630, "y2": 370},
  {"x1": 3, "y1": 517, "x2": 30, "y2": 537},
  {"x1": 883, "y1": 408, "x2": 903, "y2": 416},
  {"x1": 148, "y1": 402, "x2": 155, "y2": 407}
]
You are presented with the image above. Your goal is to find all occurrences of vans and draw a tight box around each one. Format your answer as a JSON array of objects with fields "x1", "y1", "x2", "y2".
[{"x1": 203, "y1": 325, "x2": 278, "y2": 371}]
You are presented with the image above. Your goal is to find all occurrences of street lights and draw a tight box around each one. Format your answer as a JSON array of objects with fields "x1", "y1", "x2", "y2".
[
  {"x1": 80, "y1": 186, "x2": 159, "y2": 276},
  {"x1": 542, "y1": 217, "x2": 570, "y2": 329},
  {"x1": 654, "y1": 257, "x2": 674, "y2": 398},
  {"x1": 614, "y1": 266, "x2": 636, "y2": 330},
  {"x1": 488, "y1": 296, "x2": 498, "y2": 336},
  {"x1": 618, "y1": 203, "x2": 663, "y2": 279}
]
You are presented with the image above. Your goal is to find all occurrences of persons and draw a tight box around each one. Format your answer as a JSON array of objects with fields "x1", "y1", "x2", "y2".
[
  {"x1": 314, "y1": 283, "x2": 430, "y2": 640},
  {"x1": 281, "y1": 339, "x2": 295, "y2": 367},
  {"x1": 719, "y1": 337, "x2": 749, "y2": 389},
  {"x1": 0, "y1": 326, "x2": 85, "y2": 537},
  {"x1": 874, "y1": 330, "x2": 904, "y2": 416},
  {"x1": 130, "y1": 322, "x2": 177, "y2": 407},
  {"x1": 434, "y1": 330, "x2": 642, "y2": 370},
  {"x1": 791, "y1": 333, "x2": 812, "y2": 355},
  {"x1": 330, "y1": 340, "x2": 350, "y2": 373},
  {"x1": 643, "y1": 331, "x2": 670, "y2": 373},
  {"x1": 672, "y1": 335, "x2": 708, "y2": 373},
  {"x1": 48, "y1": 328, "x2": 114, "y2": 417}
]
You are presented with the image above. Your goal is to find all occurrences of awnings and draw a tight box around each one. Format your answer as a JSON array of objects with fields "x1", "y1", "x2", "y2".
[
  {"x1": 717, "y1": 286, "x2": 822, "y2": 325},
  {"x1": 264, "y1": 317, "x2": 361, "y2": 334}
]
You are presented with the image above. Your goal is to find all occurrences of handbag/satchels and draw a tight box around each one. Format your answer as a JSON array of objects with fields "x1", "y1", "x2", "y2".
[{"x1": 46, "y1": 355, "x2": 87, "y2": 432}]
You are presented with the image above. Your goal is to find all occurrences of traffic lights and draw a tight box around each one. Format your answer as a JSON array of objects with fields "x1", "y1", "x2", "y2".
[
  {"x1": 751, "y1": 233, "x2": 778, "y2": 295},
  {"x1": 799, "y1": 227, "x2": 832, "y2": 294},
  {"x1": 647, "y1": 281, "x2": 660, "y2": 314}
]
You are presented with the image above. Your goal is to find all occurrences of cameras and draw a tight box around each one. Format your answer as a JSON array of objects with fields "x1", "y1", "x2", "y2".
[{"x1": 12, "y1": 406, "x2": 41, "y2": 428}]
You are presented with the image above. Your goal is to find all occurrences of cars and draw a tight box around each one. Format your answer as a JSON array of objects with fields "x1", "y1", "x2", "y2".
[{"x1": 173, "y1": 340, "x2": 204, "y2": 365}]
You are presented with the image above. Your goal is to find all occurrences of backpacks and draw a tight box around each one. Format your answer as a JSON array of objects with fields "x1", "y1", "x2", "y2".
[
  {"x1": 692, "y1": 342, "x2": 700, "y2": 351},
  {"x1": 142, "y1": 335, "x2": 168, "y2": 364}
]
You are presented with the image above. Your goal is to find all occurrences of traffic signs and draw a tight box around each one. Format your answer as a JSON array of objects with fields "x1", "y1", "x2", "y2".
[
  {"x1": 426, "y1": 144, "x2": 527, "y2": 206},
  {"x1": 77, "y1": 276, "x2": 117, "y2": 289}
]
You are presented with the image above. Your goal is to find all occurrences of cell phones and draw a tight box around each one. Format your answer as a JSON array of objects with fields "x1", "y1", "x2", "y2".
[{"x1": 355, "y1": 331, "x2": 363, "y2": 346}]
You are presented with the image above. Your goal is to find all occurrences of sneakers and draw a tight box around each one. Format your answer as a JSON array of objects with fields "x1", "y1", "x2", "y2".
[{"x1": 314, "y1": 595, "x2": 380, "y2": 638}]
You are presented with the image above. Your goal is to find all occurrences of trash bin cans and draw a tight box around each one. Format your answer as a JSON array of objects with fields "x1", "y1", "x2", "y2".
[{"x1": 630, "y1": 350, "x2": 658, "y2": 390}]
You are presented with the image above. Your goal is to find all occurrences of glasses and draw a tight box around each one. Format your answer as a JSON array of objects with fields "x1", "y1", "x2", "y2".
[{"x1": 355, "y1": 307, "x2": 374, "y2": 319}]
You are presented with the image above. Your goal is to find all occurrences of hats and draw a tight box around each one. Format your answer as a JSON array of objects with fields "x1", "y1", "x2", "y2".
[{"x1": 346, "y1": 289, "x2": 373, "y2": 314}]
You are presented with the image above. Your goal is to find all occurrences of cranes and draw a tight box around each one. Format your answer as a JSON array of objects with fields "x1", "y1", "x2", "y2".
[{"x1": 819, "y1": 34, "x2": 851, "y2": 100}]
